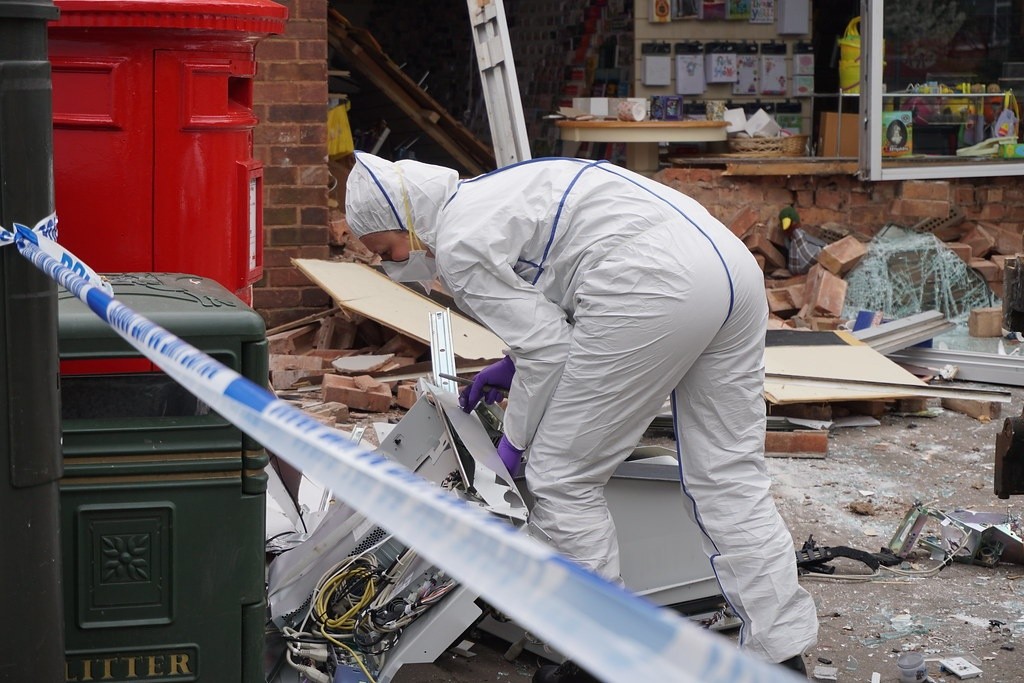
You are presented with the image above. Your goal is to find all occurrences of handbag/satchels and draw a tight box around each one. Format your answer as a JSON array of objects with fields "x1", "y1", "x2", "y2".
[
  {"x1": 964, "y1": 84, "x2": 986, "y2": 145},
  {"x1": 327, "y1": 100, "x2": 354, "y2": 160},
  {"x1": 994, "y1": 91, "x2": 1019, "y2": 138}
]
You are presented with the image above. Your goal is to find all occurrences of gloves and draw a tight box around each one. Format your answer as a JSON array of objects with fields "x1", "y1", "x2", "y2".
[
  {"x1": 459, "y1": 355, "x2": 515, "y2": 414},
  {"x1": 497, "y1": 432, "x2": 528, "y2": 479}
]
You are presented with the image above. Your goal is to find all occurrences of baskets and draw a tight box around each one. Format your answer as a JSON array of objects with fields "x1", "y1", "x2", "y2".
[
  {"x1": 839, "y1": 16, "x2": 885, "y2": 93},
  {"x1": 726, "y1": 134, "x2": 810, "y2": 156}
]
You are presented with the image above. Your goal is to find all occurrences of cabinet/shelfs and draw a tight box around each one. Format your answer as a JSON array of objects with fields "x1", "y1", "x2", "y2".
[{"x1": 364, "y1": 0, "x2": 814, "y2": 159}]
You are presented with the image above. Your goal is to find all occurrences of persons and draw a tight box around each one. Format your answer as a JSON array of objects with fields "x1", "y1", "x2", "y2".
[{"x1": 344, "y1": 150, "x2": 820, "y2": 683}]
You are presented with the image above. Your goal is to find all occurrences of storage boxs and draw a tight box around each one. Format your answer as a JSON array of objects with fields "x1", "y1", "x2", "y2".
[
  {"x1": 268, "y1": 377, "x2": 551, "y2": 683},
  {"x1": 817, "y1": 112, "x2": 859, "y2": 157},
  {"x1": 998, "y1": 143, "x2": 1024, "y2": 159},
  {"x1": 882, "y1": 111, "x2": 912, "y2": 157}
]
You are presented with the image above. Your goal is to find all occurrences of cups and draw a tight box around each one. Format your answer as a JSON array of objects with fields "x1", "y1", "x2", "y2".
[
  {"x1": 617, "y1": 101, "x2": 646, "y2": 121},
  {"x1": 706, "y1": 100, "x2": 726, "y2": 122}
]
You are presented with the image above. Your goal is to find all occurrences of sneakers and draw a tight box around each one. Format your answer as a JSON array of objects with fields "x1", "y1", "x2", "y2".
[
  {"x1": 781, "y1": 655, "x2": 807, "y2": 677},
  {"x1": 532, "y1": 660, "x2": 601, "y2": 683}
]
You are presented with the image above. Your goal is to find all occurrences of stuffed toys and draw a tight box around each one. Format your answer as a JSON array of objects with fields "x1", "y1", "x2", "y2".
[{"x1": 778, "y1": 207, "x2": 828, "y2": 276}]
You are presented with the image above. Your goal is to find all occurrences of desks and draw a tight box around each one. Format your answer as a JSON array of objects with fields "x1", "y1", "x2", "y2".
[{"x1": 555, "y1": 120, "x2": 732, "y2": 178}]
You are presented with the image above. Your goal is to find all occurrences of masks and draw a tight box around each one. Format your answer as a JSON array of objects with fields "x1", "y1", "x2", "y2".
[{"x1": 380, "y1": 225, "x2": 437, "y2": 282}]
